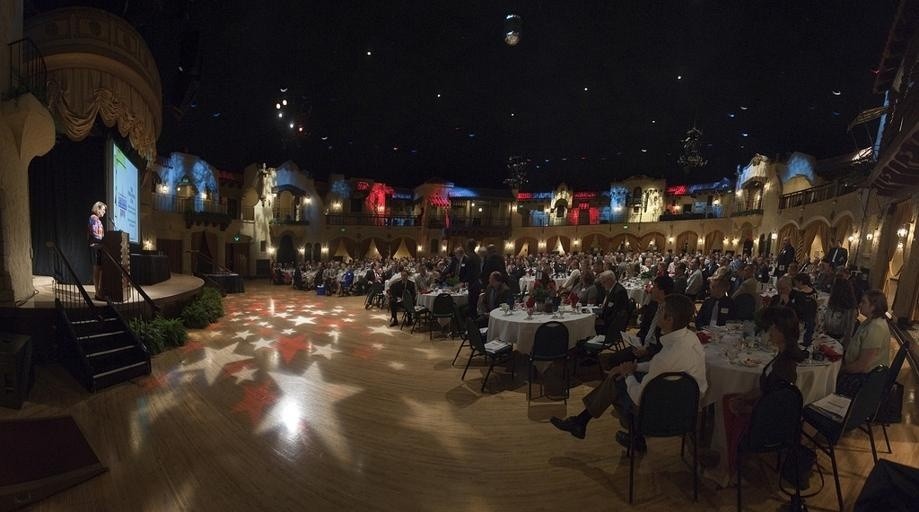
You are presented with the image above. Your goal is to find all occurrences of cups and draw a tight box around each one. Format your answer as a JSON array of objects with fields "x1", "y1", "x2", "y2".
[
  {"x1": 624, "y1": 276, "x2": 644, "y2": 289},
  {"x1": 500, "y1": 301, "x2": 595, "y2": 319},
  {"x1": 524, "y1": 267, "x2": 573, "y2": 279},
  {"x1": 762, "y1": 282, "x2": 779, "y2": 296},
  {"x1": 701, "y1": 318, "x2": 838, "y2": 369},
  {"x1": 433, "y1": 287, "x2": 468, "y2": 296}
]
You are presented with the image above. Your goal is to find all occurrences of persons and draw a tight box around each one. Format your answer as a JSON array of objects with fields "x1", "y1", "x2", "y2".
[
  {"x1": 515, "y1": 238, "x2": 890, "y2": 486},
  {"x1": 271, "y1": 238, "x2": 520, "y2": 359},
  {"x1": 87, "y1": 201, "x2": 107, "y2": 300}
]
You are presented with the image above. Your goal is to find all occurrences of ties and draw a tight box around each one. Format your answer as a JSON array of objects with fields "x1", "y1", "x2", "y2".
[{"x1": 710, "y1": 301, "x2": 720, "y2": 325}]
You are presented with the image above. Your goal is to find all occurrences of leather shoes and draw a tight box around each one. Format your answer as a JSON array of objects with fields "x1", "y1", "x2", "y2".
[
  {"x1": 616, "y1": 431, "x2": 647, "y2": 452},
  {"x1": 550, "y1": 416, "x2": 585, "y2": 439}
]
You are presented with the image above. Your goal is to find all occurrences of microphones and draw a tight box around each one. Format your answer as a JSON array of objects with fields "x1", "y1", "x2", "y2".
[{"x1": 109, "y1": 215, "x2": 117, "y2": 230}]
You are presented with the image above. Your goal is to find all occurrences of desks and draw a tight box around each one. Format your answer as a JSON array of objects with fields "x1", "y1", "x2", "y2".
[{"x1": 130, "y1": 253, "x2": 172, "y2": 287}]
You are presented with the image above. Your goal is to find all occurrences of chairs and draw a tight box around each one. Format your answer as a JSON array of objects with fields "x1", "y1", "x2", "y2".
[
  {"x1": 273, "y1": 257, "x2": 833, "y2": 340},
  {"x1": 451, "y1": 303, "x2": 910, "y2": 511}
]
